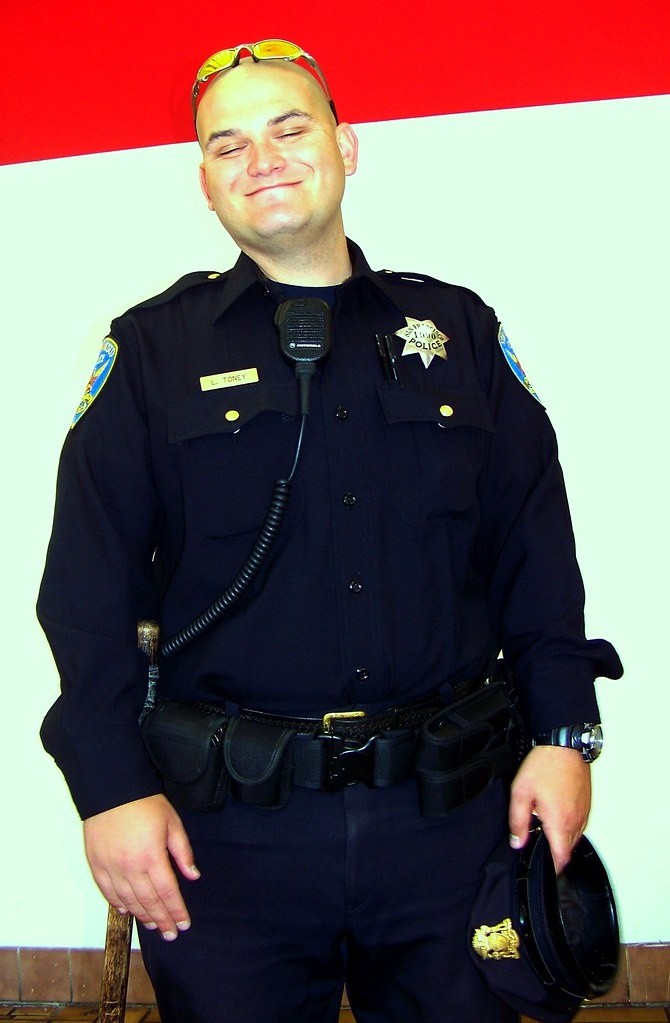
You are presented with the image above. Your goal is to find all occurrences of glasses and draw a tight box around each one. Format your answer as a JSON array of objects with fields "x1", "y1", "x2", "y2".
[{"x1": 190, "y1": 39, "x2": 341, "y2": 144}]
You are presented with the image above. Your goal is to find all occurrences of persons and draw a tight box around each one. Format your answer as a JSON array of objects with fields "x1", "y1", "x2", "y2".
[{"x1": 35, "y1": 40, "x2": 624, "y2": 1023}]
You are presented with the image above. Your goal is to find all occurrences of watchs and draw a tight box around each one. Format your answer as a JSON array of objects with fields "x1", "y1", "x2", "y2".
[{"x1": 532, "y1": 723, "x2": 604, "y2": 763}]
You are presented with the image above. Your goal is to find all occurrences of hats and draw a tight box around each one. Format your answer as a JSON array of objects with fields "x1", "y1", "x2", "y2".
[{"x1": 466, "y1": 814, "x2": 622, "y2": 1023}]
